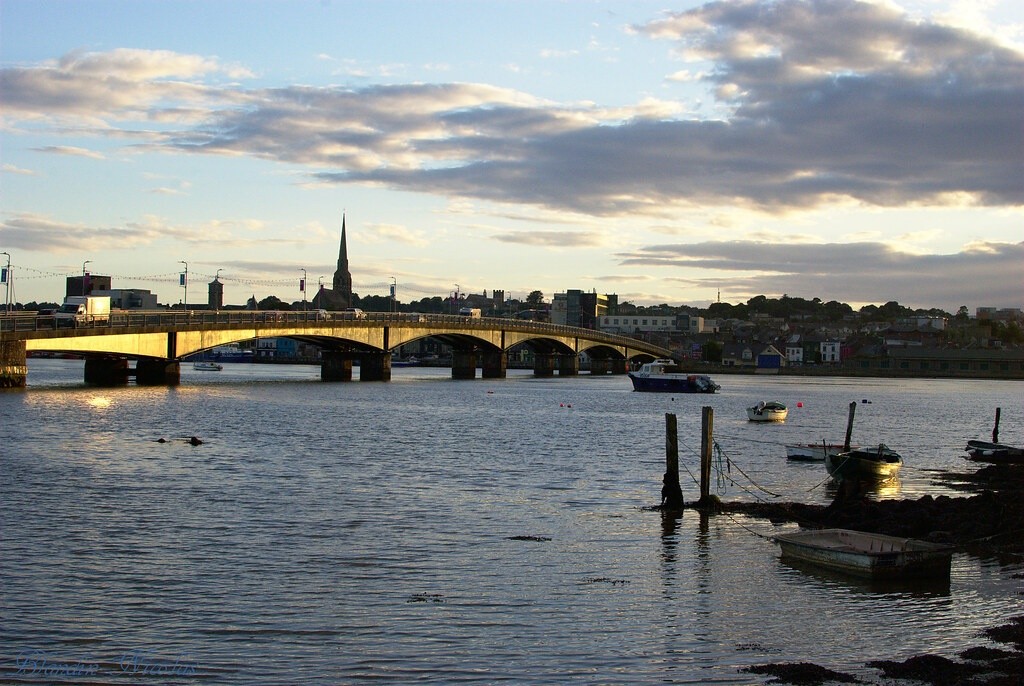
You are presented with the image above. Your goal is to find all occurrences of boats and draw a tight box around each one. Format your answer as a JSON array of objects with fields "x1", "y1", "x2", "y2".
[
  {"x1": 770, "y1": 527, "x2": 956, "y2": 583},
  {"x1": 628, "y1": 360, "x2": 721, "y2": 395},
  {"x1": 964, "y1": 439, "x2": 1024, "y2": 464},
  {"x1": 745, "y1": 399, "x2": 788, "y2": 421},
  {"x1": 784, "y1": 442, "x2": 863, "y2": 462},
  {"x1": 823, "y1": 443, "x2": 903, "y2": 480},
  {"x1": 192, "y1": 362, "x2": 224, "y2": 371}
]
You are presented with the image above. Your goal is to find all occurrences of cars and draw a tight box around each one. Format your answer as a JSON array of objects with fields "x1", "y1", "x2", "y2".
[
  {"x1": 345, "y1": 308, "x2": 368, "y2": 319},
  {"x1": 36, "y1": 309, "x2": 57, "y2": 328},
  {"x1": 307, "y1": 308, "x2": 333, "y2": 319},
  {"x1": 264, "y1": 314, "x2": 285, "y2": 319}
]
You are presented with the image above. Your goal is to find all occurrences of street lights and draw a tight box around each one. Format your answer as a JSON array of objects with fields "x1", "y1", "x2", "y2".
[
  {"x1": 390, "y1": 276, "x2": 397, "y2": 313},
  {"x1": 80, "y1": 260, "x2": 93, "y2": 295},
  {"x1": 508, "y1": 291, "x2": 511, "y2": 320},
  {"x1": 318, "y1": 275, "x2": 326, "y2": 310},
  {"x1": 216, "y1": 268, "x2": 224, "y2": 311},
  {"x1": 178, "y1": 260, "x2": 188, "y2": 321},
  {"x1": 299, "y1": 268, "x2": 309, "y2": 312}
]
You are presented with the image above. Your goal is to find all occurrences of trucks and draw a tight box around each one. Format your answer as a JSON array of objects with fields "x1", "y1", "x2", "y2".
[
  {"x1": 460, "y1": 308, "x2": 481, "y2": 321},
  {"x1": 54, "y1": 295, "x2": 111, "y2": 325}
]
